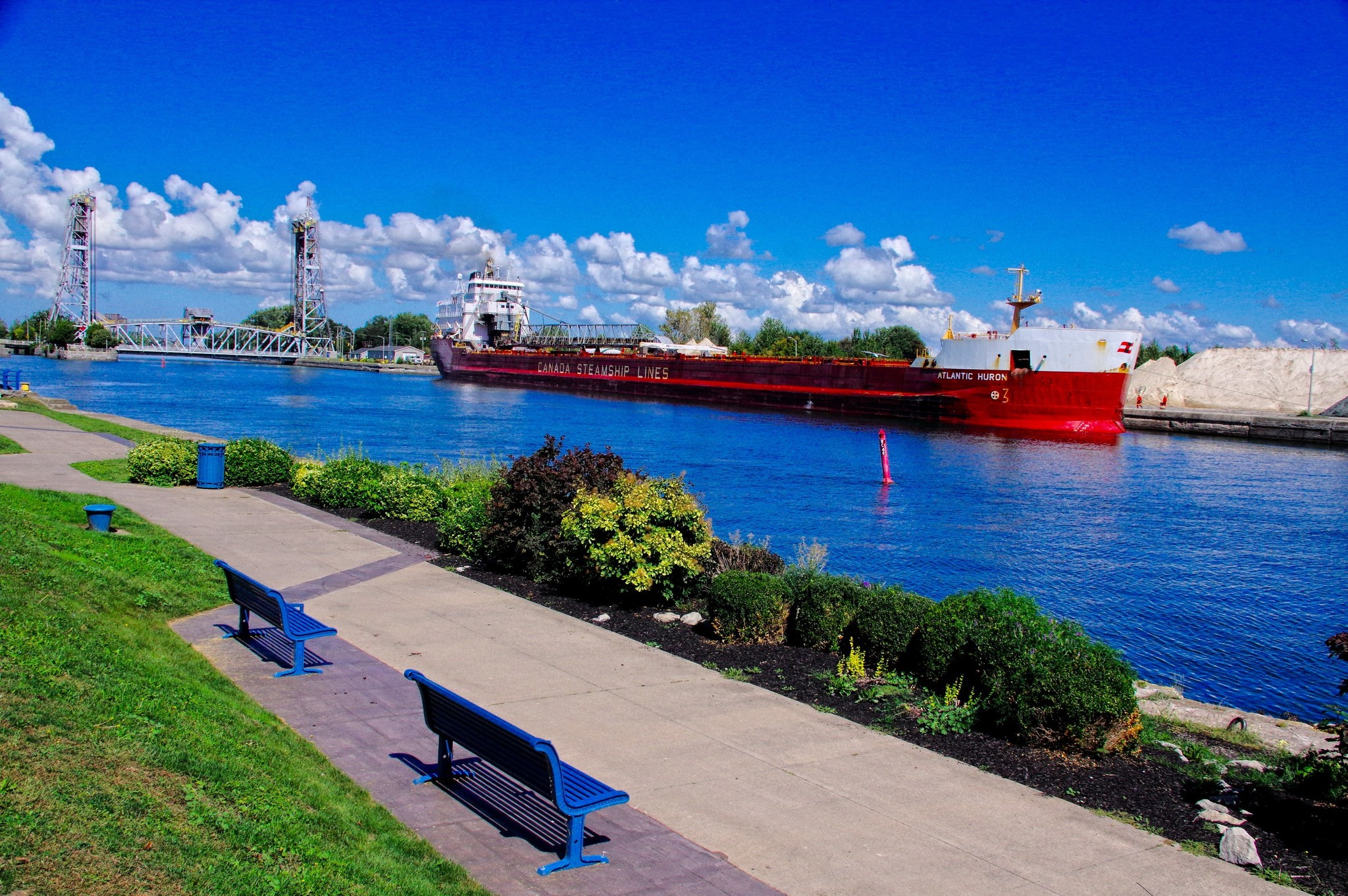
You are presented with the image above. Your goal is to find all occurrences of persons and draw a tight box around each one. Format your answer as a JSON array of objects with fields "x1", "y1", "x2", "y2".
[
  {"x1": 1136, "y1": 394, "x2": 1143, "y2": 408},
  {"x1": 732, "y1": 350, "x2": 737, "y2": 355},
  {"x1": 742, "y1": 350, "x2": 745, "y2": 355},
  {"x1": 1162, "y1": 395, "x2": 1167, "y2": 406}
]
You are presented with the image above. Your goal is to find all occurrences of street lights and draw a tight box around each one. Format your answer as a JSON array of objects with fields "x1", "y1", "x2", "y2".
[
  {"x1": 405, "y1": 337, "x2": 411, "y2": 344},
  {"x1": 336, "y1": 336, "x2": 343, "y2": 359},
  {"x1": 375, "y1": 335, "x2": 384, "y2": 360},
  {"x1": 787, "y1": 337, "x2": 800, "y2": 358},
  {"x1": 1302, "y1": 339, "x2": 1315, "y2": 418}
]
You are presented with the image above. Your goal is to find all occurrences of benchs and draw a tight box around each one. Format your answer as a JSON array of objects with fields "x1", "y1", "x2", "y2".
[
  {"x1": 404, "y1": 670, "x2": 629, "y2": 876},
  {"x1": 212, "y1": 558, "x2": 338, "y2": 677}
]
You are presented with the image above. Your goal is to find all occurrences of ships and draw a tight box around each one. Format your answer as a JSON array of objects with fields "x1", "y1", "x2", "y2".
[{"x1": 426, "y1": 251, "x2": 1141, "y2": 444}]
